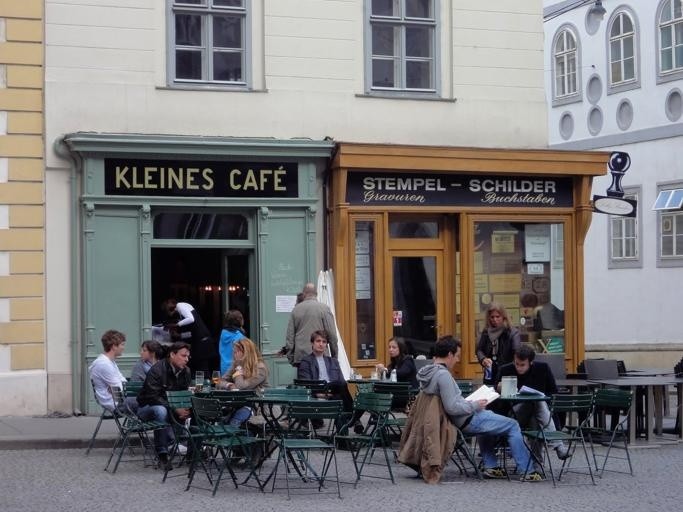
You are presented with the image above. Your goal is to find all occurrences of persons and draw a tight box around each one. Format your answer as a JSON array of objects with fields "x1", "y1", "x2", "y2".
[
  {"x1": 214, "y1": 338, "x2": 272, "y2": 468},
  {"x1": 475, "y1": 300, "x2": 520, "y2": 385},
  {"x1": 85, "y1": 328, "x2": 129, "y2": 412},
  {"x1": 493, "y1": 343, "x2": 573, "y2": 479},
  {"x1": 374, "y1": 334, "x2": 419, "y2": 446},
  {"x1": 414, "y1": 334, "x2": 545, "y2": 482},
  {"x1": 274, "y1": 292, "x2": 303, "y2": 360},
  {"x1": 126, "y1": 339, "x2": 167, "y2": 413},
  {"x1": 134, "y1": 340, "x2": 193, "y2": 470},
  {"x1": 284, "y1": 282, "x2": 338, "y2": 367},
  {"x1": 294, "y1": 327, "x2": 364, "y2": 439},
  {"x1": 159, "y1": 298, "x2": 210, "y2": 375},
  {"x1": 217, "y1": 309, "x2": 252, "y2": 378}
]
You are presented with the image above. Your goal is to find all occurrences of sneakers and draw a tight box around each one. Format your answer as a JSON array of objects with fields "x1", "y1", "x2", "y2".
[
  {"x1": 520, "y1": 471, "x2": 546, "y2": 481},
  {"x1": 158, "y1": 461, "x2": 172, "y2": 471},
  {"x1": 232, "y1": 451, "x2": 242, "y2": 463},
  {"x1": 186, "y1": 449, "x2": 208, "y2": 464},
  {"x1": 555, "y1": 448, "x2": 573, "y2": 459},
  {"x1": 481, "y1": 467, "x2": 507, "y2": 478},
  {"x1": 168, "y1": 444, "x2": 187, "y2": 455},
  {"x1": 248, "y1": 455, "x2": 260, "y2": 469}
]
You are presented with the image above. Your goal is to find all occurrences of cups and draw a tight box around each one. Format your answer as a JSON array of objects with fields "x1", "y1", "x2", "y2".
[
  {"x1": 501, "y1": 375, "x2": 518, "y2": 396},
  {"x1": 195, "y1": 371, "x2": 204, "y2": 392}
]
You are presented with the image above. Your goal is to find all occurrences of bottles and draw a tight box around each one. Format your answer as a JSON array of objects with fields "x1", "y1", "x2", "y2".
[
  {"x1": 485, "y1": 364, "x2": 491, "y2": 380},
  {"x1": 381, "y1": 369, "x2": 387, "y2": 380}
]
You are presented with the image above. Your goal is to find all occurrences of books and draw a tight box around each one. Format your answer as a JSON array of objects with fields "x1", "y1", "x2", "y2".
[{"x1": 462, "y1": 384, "x2": 501, "y2": 406}]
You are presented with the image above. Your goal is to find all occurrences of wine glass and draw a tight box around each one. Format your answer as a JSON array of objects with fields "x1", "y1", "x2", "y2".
[{"x1": 211, "y1": 370, "x2": 220, "y2": 391}]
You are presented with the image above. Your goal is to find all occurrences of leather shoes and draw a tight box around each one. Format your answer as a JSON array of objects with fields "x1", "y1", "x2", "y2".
[
  {"x1": 354, "y1": 423, "x2": 364, "y2": 434},
  {"x1": 338, "y1": 442, "x2": 360, "y2": 450}
]
